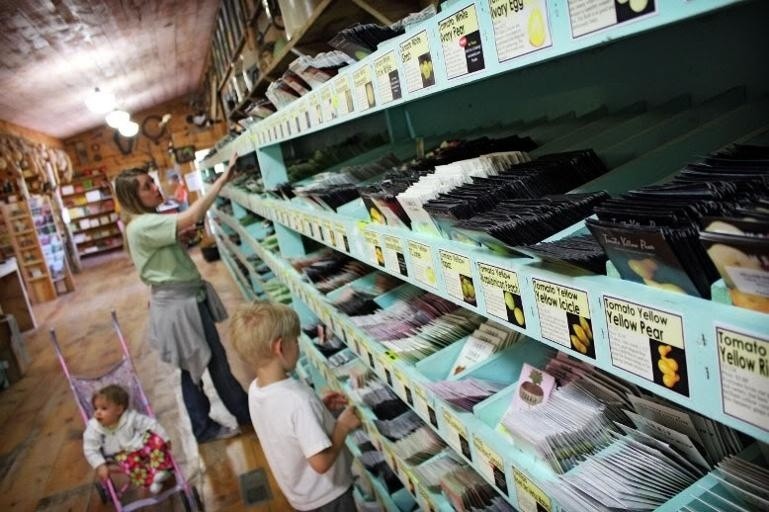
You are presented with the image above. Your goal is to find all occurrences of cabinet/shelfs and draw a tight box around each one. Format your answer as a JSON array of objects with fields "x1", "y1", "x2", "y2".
[{"x1": 0, "y1": 167, "x2": 125, "y2": 304}]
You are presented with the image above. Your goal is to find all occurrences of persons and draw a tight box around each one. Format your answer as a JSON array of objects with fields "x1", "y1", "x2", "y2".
[
  {"x1": 113, "y1": 151, "x2": 254, "y2": 443},
  {"x1": 166, "y1": 174, "x2": 189, "y2": 211},
  {"x1": 81, "y1": 383, "x2": 174, "y2": 497},
  {"x1": 226, "y1": 298, "x2": 361, "y2": 512}
]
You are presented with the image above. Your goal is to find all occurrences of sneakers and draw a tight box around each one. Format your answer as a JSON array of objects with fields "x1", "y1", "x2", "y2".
[
  {"x1": 148, "y1": 470, "x2": 174, "y2": 496},
  {"x1": 204, "y1": 423, "x2": 241, "y2": 441}
]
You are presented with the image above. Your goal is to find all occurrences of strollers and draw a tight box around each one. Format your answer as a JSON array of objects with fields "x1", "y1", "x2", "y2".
[{"x1": 46, "y1": 305, "x2": 204, "y2": 511}]
